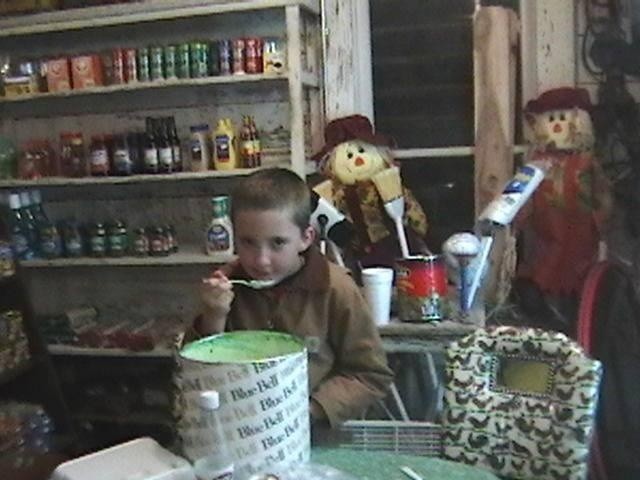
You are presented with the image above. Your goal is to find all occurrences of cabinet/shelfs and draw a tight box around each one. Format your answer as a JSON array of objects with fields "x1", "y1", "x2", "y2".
[{"x1": 0, "y1": 0, "x2": 327, "y2": 480}]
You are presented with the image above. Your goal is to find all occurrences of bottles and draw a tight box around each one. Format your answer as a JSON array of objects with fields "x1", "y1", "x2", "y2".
[
  {"x1": 193, "y1": 391, "x2": 233, "y2": 480},
  {"x1": 143, "y1": 116, "x2": 183, "y2": 173},
  {"x1": 6, "y1": 189, "x2": 51, "y2": 259},
  {"x1": 239, "y1": 115, "x2": 262, "y2": 169},
  {"x1": 205, "y1": 195, "x2": 234, "y2": 257}
]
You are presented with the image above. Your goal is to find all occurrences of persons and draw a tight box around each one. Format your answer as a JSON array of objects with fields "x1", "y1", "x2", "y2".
[
  {"x1": 309, "y1": 111, "x2": 427, "y2": 285},
  {"x1": 183, "y1": 167, "x2": 396, "y2": 439},
  {"x1": 511, "y1": 86, "x2": 608, "y2": 344}
]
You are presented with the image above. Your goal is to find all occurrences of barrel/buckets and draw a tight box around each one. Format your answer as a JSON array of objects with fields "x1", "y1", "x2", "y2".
[{"x1": 172, "y1": 328, "x2": 310, "y2": 480}]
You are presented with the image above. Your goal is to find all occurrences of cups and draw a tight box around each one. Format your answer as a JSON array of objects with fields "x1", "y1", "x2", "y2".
[{"x1": 361, "y1": 268, "x2": 393, "y2": 327}]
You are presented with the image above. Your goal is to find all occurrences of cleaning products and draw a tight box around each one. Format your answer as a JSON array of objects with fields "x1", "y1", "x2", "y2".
[{"x1": 189, "y1": 118, "x2": 237, "y2": 174}]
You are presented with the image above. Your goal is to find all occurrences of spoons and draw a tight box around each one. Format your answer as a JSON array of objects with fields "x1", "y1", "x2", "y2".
[{"x1": 201, "y1": 279, "x2": 274, "y2": 290}]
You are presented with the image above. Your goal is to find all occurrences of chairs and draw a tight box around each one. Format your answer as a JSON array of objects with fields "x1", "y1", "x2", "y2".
[{"x1": 433, "y1": 325, "x2": 603, "y2": 479}]
